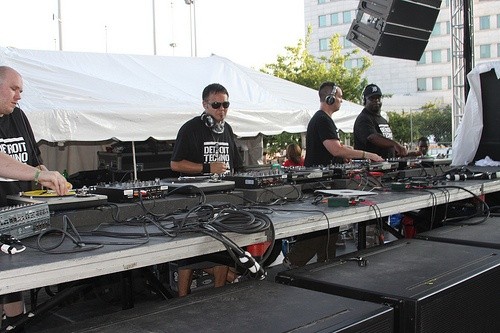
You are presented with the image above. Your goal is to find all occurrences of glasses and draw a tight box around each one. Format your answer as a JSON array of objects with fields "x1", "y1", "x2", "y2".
[{"x1": 207, "y1": 101, "x2": 230, "y2": 109}]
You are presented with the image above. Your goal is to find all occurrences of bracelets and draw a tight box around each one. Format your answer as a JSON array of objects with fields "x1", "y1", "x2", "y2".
[
  {"x1": 34, "y1": 169, "x2": 43, "y2": 188},
  {"x1": 362, "y1": 150, "x2": 367, "y2": 160}
]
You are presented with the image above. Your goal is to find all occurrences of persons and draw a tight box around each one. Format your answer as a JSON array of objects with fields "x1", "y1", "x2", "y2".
[
  {"x1": 1, "y1": 66, "x2": 69, "y2": 324},
  {"x1": 284, "y1": 144, "x2": 305, "y2": 169},
  {"x1": 407, "y1": 136, "x2": 432, "y2": 159},
  {"x1": 353, "y1": 84, "x2": 406, "y2": 247},
  {"x1": 283, "y1": 82, "x2": 385, "y2": 269},
  {"x1": 169, "y1": 84, "x2": 244, "y2": 296}
]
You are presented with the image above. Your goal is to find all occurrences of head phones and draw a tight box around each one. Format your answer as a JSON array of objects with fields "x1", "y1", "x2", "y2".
[
  {"x1": 325, "y1": 85, "x2": 337, "y2": 105},
  {"x1": 201, "y1": 111, "x2": 215, "y2": 128},
  {"x1": 359, "y1": 85, "x2": 367, "y2": 106}
]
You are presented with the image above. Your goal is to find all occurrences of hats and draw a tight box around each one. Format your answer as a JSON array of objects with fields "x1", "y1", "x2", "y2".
[{"x1": 364, "y1": 83, "x2": 382, "y2": 99}]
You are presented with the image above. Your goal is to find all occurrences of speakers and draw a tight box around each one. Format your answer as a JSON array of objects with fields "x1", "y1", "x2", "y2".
[
  {"x1": 414, "y1": 212, "x2": 500, "y2": 249},
  {"x1": 275, "y1": 237, "x2": 500, "y2": 333},
  {"x1": 28, "y1": 277, "x2": 395, "y2": 333}
]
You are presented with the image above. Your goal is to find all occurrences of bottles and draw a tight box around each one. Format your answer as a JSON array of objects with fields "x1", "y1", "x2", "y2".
[{"x1": 62, "y1": 169, "x2": 67, "y2": 178}]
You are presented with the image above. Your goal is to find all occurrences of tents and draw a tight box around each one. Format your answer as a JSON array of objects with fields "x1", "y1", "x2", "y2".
[{"x1": 1, "y1": 48, "x2": 395, "y2": 190}]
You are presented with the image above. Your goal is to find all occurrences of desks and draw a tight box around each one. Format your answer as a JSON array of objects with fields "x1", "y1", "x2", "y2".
[{"x1": 0, "y1": 177, "x2": 499, "y2": 296}]
